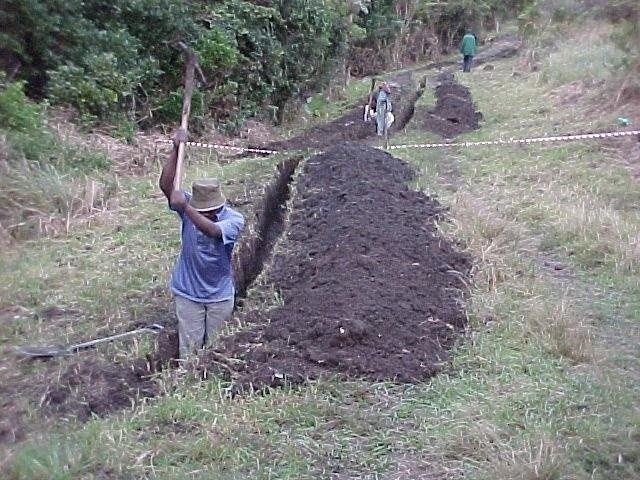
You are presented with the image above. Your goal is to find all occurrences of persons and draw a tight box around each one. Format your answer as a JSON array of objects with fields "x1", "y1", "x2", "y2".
[
  {"x1": 367, "y1": 94, "x2": 395, "y2": 135},
  {"x1": 157, "y1": 129, "x2": 245, "y2": 368},
  {"x1": 376, "y1": 81, "x2": 391, "y2": 136},
  {"x1": 459, "y1": 28, "x2": 476, "y2": 73}
]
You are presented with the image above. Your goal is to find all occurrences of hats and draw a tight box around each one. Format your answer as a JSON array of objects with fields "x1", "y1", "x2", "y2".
[{"x1": 187, "y1": 178, "x2": 226, "y2": 212}]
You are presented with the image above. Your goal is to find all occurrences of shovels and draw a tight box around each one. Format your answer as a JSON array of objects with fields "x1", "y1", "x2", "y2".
[{"x1": 19, "y1": 323, "x2": 165, "y2": 358}]
